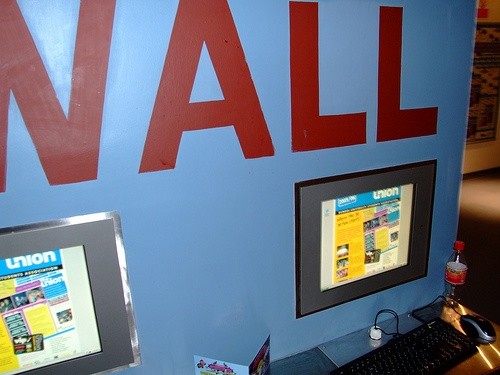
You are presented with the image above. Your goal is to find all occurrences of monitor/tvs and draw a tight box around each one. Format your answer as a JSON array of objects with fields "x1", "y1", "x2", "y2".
[
  {"x1": 0, "y1": 244, "x2": 103, "y2": 375},
  {"x1": 320, "y1": 182, "x2": 416, "y2": 293}
]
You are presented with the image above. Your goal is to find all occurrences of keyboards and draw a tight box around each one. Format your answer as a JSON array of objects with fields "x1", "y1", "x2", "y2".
[{"x1": 328, "y1": 316, "x2": 478, "y2": 375}]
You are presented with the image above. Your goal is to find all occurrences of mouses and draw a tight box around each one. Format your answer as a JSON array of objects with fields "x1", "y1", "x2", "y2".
[{"x1": 459, "y1": 314, "x2": 497, "y2": 344}]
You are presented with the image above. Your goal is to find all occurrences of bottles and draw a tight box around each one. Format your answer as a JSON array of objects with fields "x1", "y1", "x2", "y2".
[{"x1": 444, "y1": 241, "x2": 469, "y2": 308}]
